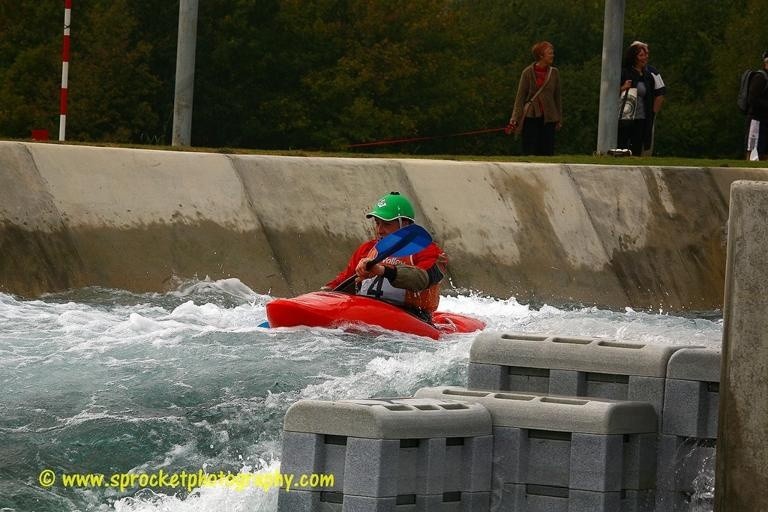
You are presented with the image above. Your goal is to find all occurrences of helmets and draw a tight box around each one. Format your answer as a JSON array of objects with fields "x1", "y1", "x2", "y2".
[{"x1": 366, "y1": 192, "x2": 415, "y2": 222}]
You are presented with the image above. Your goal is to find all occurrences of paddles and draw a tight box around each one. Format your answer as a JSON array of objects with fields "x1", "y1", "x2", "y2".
[{"x1": 259, "y1": 223, "x2": 432, "y2": 329}]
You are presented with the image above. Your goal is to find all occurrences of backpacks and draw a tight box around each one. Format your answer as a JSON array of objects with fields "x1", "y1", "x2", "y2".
[{"x1": 736, "y1": 69, "x2": 767, "y2": 113}]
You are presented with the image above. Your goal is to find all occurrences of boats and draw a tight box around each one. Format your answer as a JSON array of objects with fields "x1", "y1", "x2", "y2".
[{"x1": 264, "y1": 289, "x2": 488, "y2": 339}]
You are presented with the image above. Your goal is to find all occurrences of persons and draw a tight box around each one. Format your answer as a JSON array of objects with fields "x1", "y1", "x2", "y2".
[
  {"x1": 510, "y1": 41, "x2": 562, "y2": 154},
  {"x1": 319, "y1": 191, "x2": 448, "y2": 324},
  {"x1": 744, "y1": 54, "x2": 768, "y2": 160},
  {"x1": 630, "y1": 40, "x2": 666, "y2": 156},
  {"x1": 617, "y1": 45, "x2": 655, "y2": 156}
]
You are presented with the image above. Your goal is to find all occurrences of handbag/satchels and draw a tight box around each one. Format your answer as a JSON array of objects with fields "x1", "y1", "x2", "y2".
[
  {"x1": 513, "y1": 102, "x2": 528, "y2": 141},
  {"x1": 619, "y1": 88, "x2": 638, "y2": 121}
]
